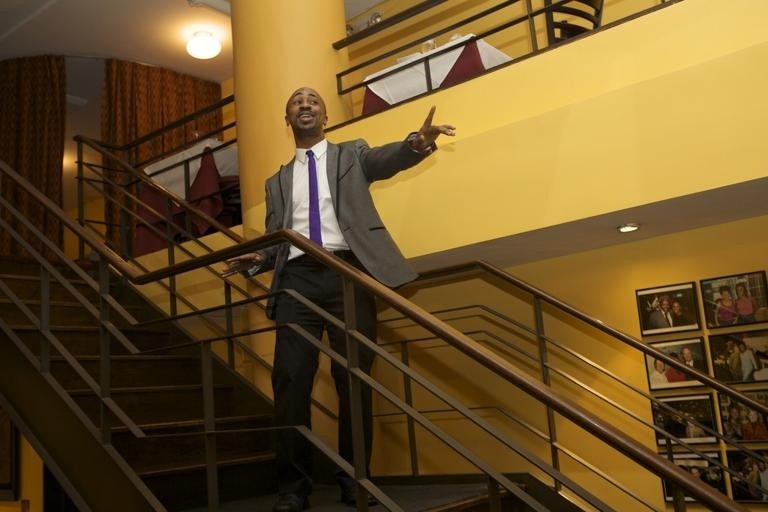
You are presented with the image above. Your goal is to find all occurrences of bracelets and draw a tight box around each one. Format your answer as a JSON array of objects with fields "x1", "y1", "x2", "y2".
[{"x1": 414, "y1": 141, "x2": 435, "y2": 153}]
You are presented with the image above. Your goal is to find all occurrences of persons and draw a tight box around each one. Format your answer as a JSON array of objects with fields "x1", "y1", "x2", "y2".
[
  {"x1": 220, "y1": 85, "x2": 457, "y2": 512},
  {"x1": 643, "y1": 282, "x2": 768, "y2": 504}
]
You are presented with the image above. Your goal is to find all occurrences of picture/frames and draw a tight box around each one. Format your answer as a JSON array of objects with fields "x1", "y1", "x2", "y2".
[{"x1": 635, "y1": 270, "x2": 766, "y2": 511}]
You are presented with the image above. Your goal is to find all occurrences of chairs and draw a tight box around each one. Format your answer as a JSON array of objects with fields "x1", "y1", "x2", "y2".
[{"x1": 544, "y1": 0, "x2": 605, "y2": 48}]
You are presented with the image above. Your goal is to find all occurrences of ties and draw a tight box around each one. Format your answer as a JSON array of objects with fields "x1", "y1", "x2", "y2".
[{"x1": 306, "y1": 150, "x2": 322, "y2": 248}]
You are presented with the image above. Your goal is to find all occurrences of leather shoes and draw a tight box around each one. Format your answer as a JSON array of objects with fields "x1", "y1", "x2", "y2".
[
  {"x1": 272, "y1": 492, "x2": 310, "y2": 512},
  {"x1": 341, "y1": 484, "x2": 377, "y2": 506}
]
